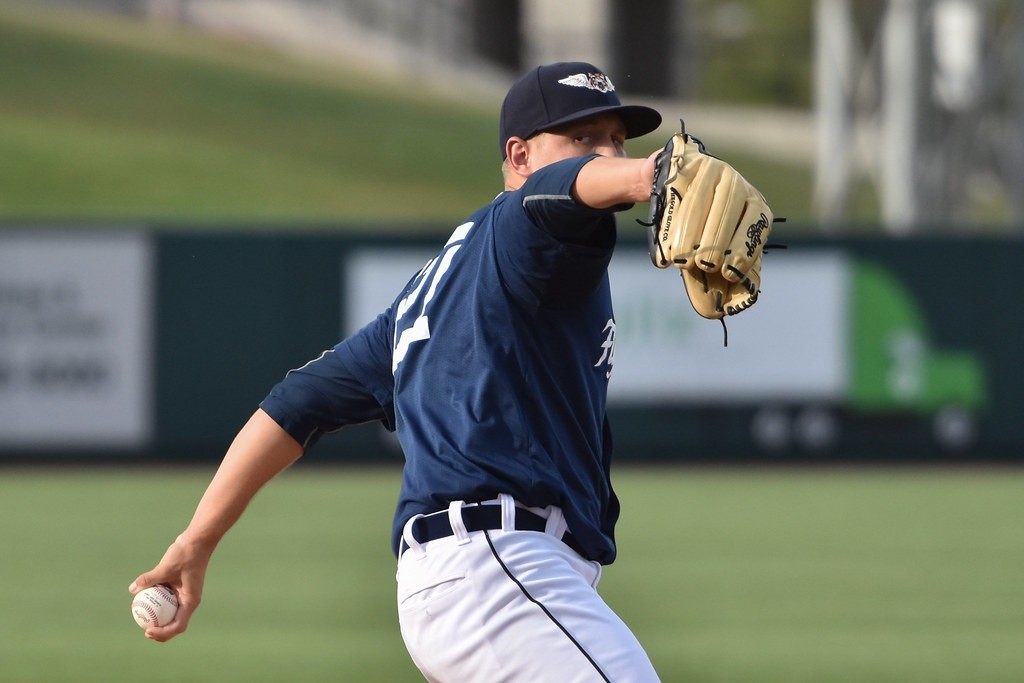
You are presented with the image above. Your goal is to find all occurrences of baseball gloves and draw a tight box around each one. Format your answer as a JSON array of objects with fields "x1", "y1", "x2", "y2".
[{"x1": 636, "y1": 119, "x2": 788, "y2": 350}]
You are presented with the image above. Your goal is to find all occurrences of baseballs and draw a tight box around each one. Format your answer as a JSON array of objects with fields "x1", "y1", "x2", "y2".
[{"x1": 132, "y1": 584, "x2": 178, "y2": 630}]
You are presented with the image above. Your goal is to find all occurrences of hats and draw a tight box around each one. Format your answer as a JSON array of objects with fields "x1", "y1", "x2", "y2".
[{"x1": 499, "y1": 63, "x2": 662, "y2": 162}]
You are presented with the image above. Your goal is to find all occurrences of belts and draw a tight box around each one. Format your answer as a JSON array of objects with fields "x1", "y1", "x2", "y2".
[{"x1": 400, "y1": 505, "x2": 591, "y2": 563}]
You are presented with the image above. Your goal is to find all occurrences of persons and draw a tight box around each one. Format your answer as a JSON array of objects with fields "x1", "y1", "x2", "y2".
[{"x1": 128, "y1": 62, "x2": 776, "y2": 683}]
